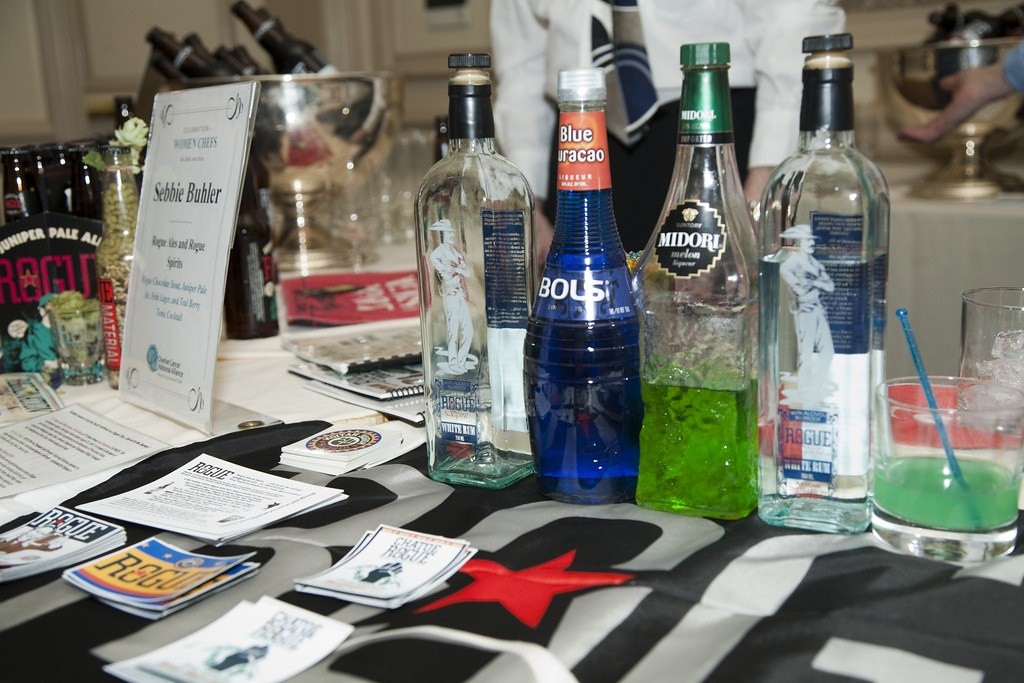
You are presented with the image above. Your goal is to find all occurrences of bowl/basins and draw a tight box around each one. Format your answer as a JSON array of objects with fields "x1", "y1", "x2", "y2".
[
  {"x1": 873, "y1": 36, "x2": 1024, "y2": 202},
  {"x1": 173, "y1": 72, "x2": 403, "y2": 269}
]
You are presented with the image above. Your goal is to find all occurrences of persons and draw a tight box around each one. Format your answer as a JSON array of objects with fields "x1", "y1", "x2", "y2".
[
  {"x1": 490, "y1": 0, "x2": 848, "y2": 312},
  {"x1": 895, "y1": 40, "x2": 1024, "y2": 141}
]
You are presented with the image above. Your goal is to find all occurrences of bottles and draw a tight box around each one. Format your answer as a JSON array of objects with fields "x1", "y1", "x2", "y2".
[
  {"x1": 413, "y1": 53, "x2": 541, "y2": 493},
  {"x1": 111, "y1": 96, "x2": 143, "y2": 191},
  {"x1": 627, "y1": 37, "x2": 763, "y2": 520},
  {"x1": 522, "y1": 68, "x2": 645, "y2": 507},
  {"x1": 751, "y1": 33, "x2": 892, "y2": 536},
  {"x1": 95, "y1": 146, "x2": 139, "y2": 391},
  {"x1": 223, "y1": 158, "x2": 280, "y2": 339},
  {"x1": 0, "y1": 142, "x2": 97, "y2": 223},
  {"x1": 142, "y1": 0, "x2": 337, "y2": 76}
]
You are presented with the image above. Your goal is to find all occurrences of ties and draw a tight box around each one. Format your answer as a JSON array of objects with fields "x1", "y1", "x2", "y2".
[{"x1": 591, "y1": 0, "x2": 662, "y2": 145}]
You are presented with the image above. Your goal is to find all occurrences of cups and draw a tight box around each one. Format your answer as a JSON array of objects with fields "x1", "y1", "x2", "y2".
[
  {"x1": 874, "y1": 375, "x2": 1024, "y2": 568},
  {"x1": 46, "y1": 305, "x2": 105, "y2": 385},
  {"x1": 957, "y1": 287, "x2": 1024, "y2": 432}
]
